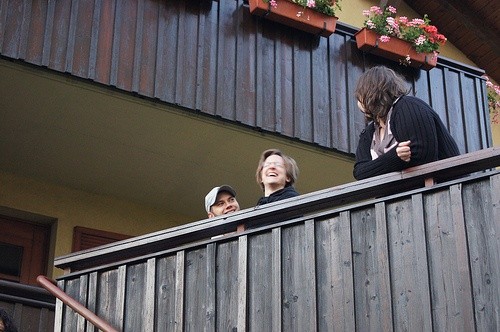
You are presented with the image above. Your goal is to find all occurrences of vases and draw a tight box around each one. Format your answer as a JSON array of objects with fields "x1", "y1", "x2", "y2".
[
  {"x1": 249, "y1": 0, "x2": 339, "y2": 38},
  {"x1": 354, "y1": 27, "x2": 439, "y2": 72}
]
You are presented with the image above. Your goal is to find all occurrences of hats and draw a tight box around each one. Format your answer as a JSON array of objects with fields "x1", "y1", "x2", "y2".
[{"x1": 205, "y1": 185, "x2": 236, "y2": 213}]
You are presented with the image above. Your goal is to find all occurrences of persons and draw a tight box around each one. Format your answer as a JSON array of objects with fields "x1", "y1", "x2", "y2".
[
  {"x1": 245, "y1": 149, "x2": 304, "y2": 230},
  {"x1": 353, "y1": 66, "x2": 471, "y2": 199},
  {"x1": 205, "y1": 185, "x2": 240, "y2": 240}
]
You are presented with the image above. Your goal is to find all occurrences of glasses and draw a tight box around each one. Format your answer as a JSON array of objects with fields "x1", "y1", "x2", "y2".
[{"x1": 261, "y1": 162, "x2": 287, "y2": 168}]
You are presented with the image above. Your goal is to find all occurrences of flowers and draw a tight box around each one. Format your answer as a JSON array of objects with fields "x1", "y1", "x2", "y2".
[
  {"x1": 270, "y1": 0, "x2": 342, "y2": 17},
  {"x1": 363, "y1": 6, "x2": 447, "y2": 54},
  {"x1": 481, "y1": 75, "x2": 500, "y2": 124}
]
